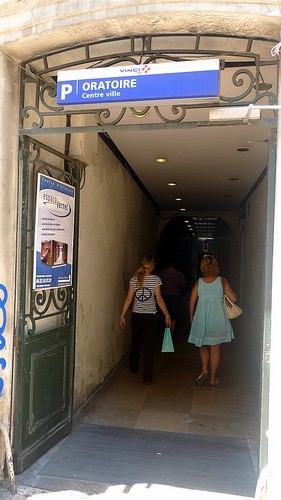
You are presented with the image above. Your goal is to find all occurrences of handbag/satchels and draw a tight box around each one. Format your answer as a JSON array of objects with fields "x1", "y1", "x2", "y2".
[
  {"x1": 161, "y1": 325, "x2": 175, "y2": 352},
  {"x1": 223, "y1": 294, "x2": 243, "y2": 319}
]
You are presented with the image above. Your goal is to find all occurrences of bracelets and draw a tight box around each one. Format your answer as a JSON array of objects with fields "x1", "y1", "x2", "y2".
[
  {"x1": 119, "y1": 315, "x2": 125, "y2": 319},
  {"x1": 165, "y1": 315, "x2": 171, "y2": 320}
]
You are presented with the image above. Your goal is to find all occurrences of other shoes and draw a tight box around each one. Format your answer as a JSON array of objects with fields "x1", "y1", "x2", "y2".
[
  {"x1": 195, "y1": 369, "x2": 208, "y2": 381},
  {"x1": 210, "y1": 378, "x2": 221, "y2": 386}
]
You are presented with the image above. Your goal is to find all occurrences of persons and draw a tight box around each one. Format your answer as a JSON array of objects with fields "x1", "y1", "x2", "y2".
[
  {"x1": 158, "y1": 259, "x2": 186, "y2": 343},
  {"x1": 118, "y1": 253, "x2": 172, "y2": 385},
  {"x1": 189, "y1": 254, "x2": 238, "y2": 386}
]
